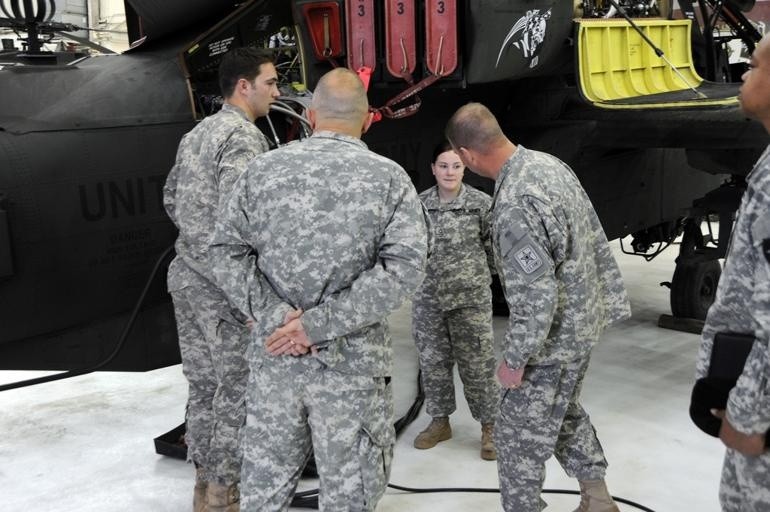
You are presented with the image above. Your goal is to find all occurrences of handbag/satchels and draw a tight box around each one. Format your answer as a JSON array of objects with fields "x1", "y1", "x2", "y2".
[{"x1": 690, "y1": 332, "x2": 770, "y2": 447}]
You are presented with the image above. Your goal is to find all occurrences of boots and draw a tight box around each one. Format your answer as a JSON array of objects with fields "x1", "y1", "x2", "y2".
[
  {"x1": 414, "y1": 417, "x2": 452, "y2": 449},
  {"x1": 480, "y1": 424, "x2": 497, "y2": 460},
  {"x1": 193, "y1": 480, "x2": 240, "y2": 512},
  {"x1": 572, "y1": 479, "x2": 619, "y2": 512}
]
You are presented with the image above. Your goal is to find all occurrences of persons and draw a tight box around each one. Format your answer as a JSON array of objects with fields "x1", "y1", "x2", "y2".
[
  {"x1": 444, "y1": 99, "x2": 626, "y2": 512},
  {"x1": 692, "y1": 30, "x2": 770, "y2": 510},
  {"x1": 158, "y1": 42, "x2": 281, "y2": 511},
  {"x1": 207, "y1": 61, "x2": 427, "y2": 512},
  {"x1": 405, "y1": 145, "x2": 509, "y2": 462}
]
